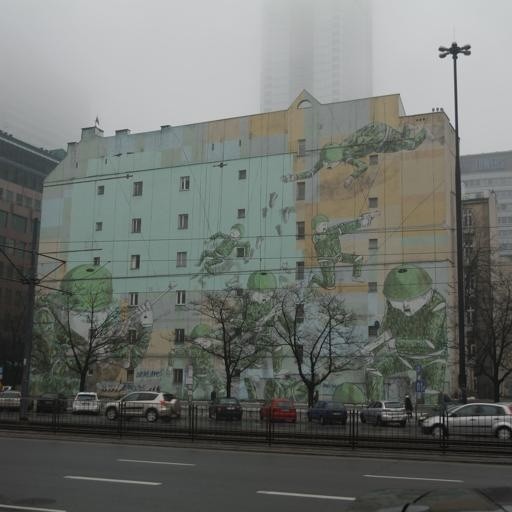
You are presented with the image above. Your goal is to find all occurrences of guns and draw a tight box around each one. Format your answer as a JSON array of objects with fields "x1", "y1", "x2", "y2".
[
  {"x1": 361, "y1": 208, "x2": 382, "y2": 226},
  {"x1": 91, "y1": 280, "x2": 177, "y2": 349},
  {"x1": 323, "y1": 329, "x2": 391, "y2": 372}
]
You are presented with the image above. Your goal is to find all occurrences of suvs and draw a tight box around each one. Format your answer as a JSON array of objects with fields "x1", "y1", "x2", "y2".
[
  {"x1": 103, "y1": 391, "x2": 181, "y2": 423},
  {"x1": 421, "y1": 403, "x2": 512, "y2": 440}
]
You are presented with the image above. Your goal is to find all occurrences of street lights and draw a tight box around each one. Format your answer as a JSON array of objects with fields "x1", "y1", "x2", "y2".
[{"x1": 438, "y1": 40, "x2": 472, "y2": 403}]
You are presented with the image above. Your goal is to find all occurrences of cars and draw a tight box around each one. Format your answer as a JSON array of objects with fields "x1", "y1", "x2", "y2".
[
  {"x1": 209, "y1": 396, "x2": 243, "y2": 421},
  {"x1": 360, "y1": 401, "x2": 408, "y2": 428},
  {"x1": 0, "y1": 390, "x2": 21, "y2": 408},
  {"x1": 259, "y1": 398, "x2": 297, "y2": 422},
  {"x1": 419, "y1": 403, "x2": 462, "y2": 427},
  {"x1": 306, "y1": 402, "x2": 348, "y2": 426},
  {"x1": 71, "y1": 391, "x2": 101, "y2": 416},
  {"x1": 36, "y1": 391, "x2": 69, "y2": 411}
]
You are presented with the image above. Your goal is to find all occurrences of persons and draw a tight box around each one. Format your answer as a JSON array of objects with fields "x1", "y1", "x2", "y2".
[
  {"x1": 404, "y1": 394, "x2": 414, "y2": 417},
  {"x1": 314, "y1": 390, "x2": 319, "y2": 402}
]
